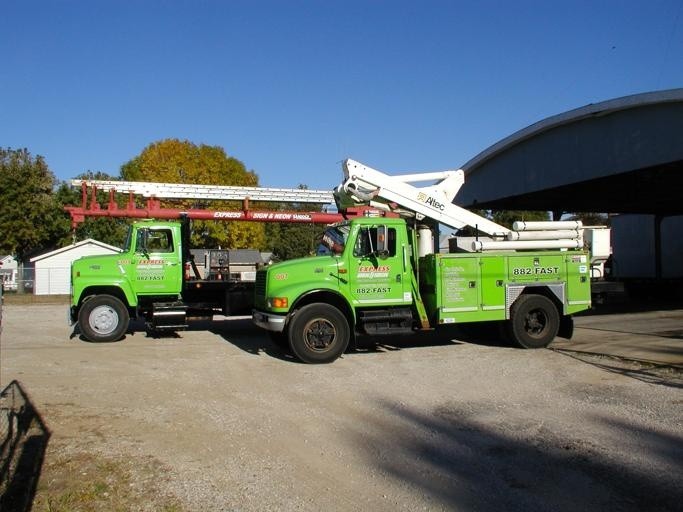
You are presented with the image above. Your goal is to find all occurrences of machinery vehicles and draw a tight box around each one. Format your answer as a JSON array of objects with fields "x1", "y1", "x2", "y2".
[
  {"x1": 61, "y1": 176, "x2": 402, "y2": 345},
  {"x1": 253, "y1": 154, "x2": 615, "y2": 364}
]
